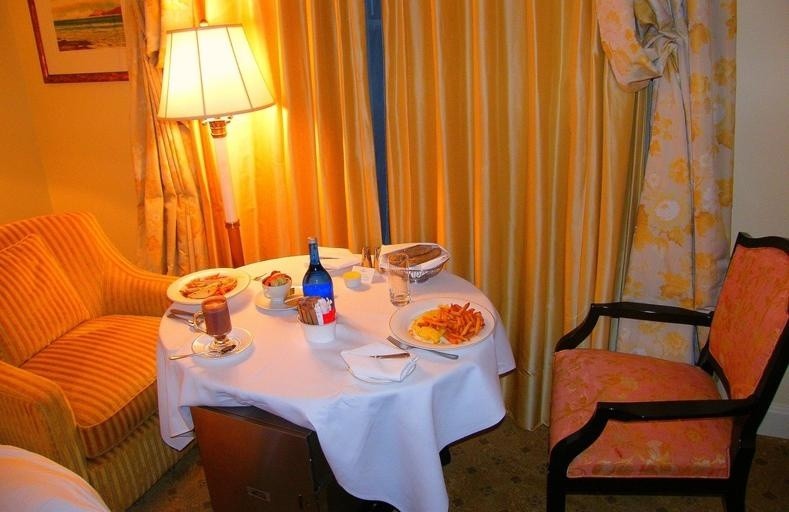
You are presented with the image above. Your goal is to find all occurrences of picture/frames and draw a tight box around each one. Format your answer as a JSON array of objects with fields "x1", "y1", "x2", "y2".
[{"x1": 27, "y1": 0, "x2": 129, "y2": 84}]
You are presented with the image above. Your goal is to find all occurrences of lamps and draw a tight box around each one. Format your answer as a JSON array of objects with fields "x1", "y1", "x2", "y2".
[{"x1": 157, "y1": 19, "x2": 277, "y2": 269}]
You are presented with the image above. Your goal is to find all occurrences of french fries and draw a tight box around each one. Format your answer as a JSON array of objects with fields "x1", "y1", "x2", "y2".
[{"x1": 414, "y1": 301, "x2": 486, "y2": 345}]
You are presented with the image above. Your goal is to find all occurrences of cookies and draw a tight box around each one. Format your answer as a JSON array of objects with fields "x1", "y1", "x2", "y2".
[{"x1": 296, "y1": 296, "x2": 333, "y2": 325}]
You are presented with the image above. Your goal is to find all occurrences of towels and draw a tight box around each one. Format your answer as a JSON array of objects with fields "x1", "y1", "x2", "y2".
[
  {"x1": 340, "y1": 343, "x2": 418, "y2": 384},
  {"x1": 305, "y1": 246, "x2": 362, "y2": 271}
]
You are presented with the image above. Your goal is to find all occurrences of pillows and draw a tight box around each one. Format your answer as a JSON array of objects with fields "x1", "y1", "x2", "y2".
[{"x1": 0, "y1": 233, "x2": 89, "y2": 367}]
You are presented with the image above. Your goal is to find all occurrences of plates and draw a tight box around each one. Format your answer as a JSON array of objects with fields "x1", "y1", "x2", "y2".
[
  {"x1": 167, "y1": 267, "x2": 251, "y2": 305},
  {"x1": 192, "y1": 330, "x2": 253, "y2": 361},
  {"x1": 389, "y1": 298, "x2": 496, "y2": 350},
  {"x1": 254, "y1": 287, "x2": 306, "y2": 312}
]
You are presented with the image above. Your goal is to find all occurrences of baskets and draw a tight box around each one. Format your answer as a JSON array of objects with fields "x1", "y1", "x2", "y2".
[{"x1": 384, "y1": 258, "x2": 450, "y2": 282}]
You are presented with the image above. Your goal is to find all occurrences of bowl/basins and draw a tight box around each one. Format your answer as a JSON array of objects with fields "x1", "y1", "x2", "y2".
[
  {"x1": 297, "y1": 314, "x2": 338, "y2": 345},
  {"x1": 384, "y1": 267, "x2": 447, "y2": 284}
]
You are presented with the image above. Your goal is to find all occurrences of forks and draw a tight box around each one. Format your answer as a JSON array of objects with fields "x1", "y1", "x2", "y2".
[{"x1": 386, "y1": 336, "x2": 459, "y2": 360}]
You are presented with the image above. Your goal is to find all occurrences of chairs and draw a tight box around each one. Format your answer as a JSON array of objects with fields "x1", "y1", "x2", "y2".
[
  {"x1": 0, "y1": 211, "x2": 197, "y2": 512},
  {"x1": 547, "y1": 232, "x2": 789, "y2": 512}
]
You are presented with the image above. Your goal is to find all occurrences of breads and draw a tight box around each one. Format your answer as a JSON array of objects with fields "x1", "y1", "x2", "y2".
[{"x1": 386, "y1": 245, "x2": 442, "y2": 268}]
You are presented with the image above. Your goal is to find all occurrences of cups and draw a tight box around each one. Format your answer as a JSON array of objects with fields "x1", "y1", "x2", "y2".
[
  {"x1": 193, "y1": 296, "x2": 233, "y2": 350},
  {"x1": 261, "y1": 273, "x2": 293, "y2": 304},
  {"x1": 344, "y1": 272, "x2": 362, "y2": 288},
  {"x1": 387, "y1": 253, "x2": 412, "y2": 307}
]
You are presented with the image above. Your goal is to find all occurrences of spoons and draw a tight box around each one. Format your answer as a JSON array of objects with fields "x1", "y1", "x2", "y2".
[
  {"x1": 167, "y1": 345, "x2": 237, "y2": 361},
  {"x1": 166, "y1": 314, "x2": 204, "y2": 328}
]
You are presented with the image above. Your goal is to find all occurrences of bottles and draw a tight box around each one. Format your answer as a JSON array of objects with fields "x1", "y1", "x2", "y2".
[
  {"x1": 301, "y1": 237, "x2": 334, "y2": 305},
  {"x1": 361, "y1": 246, "x2": 383, "y2": 272}
]
